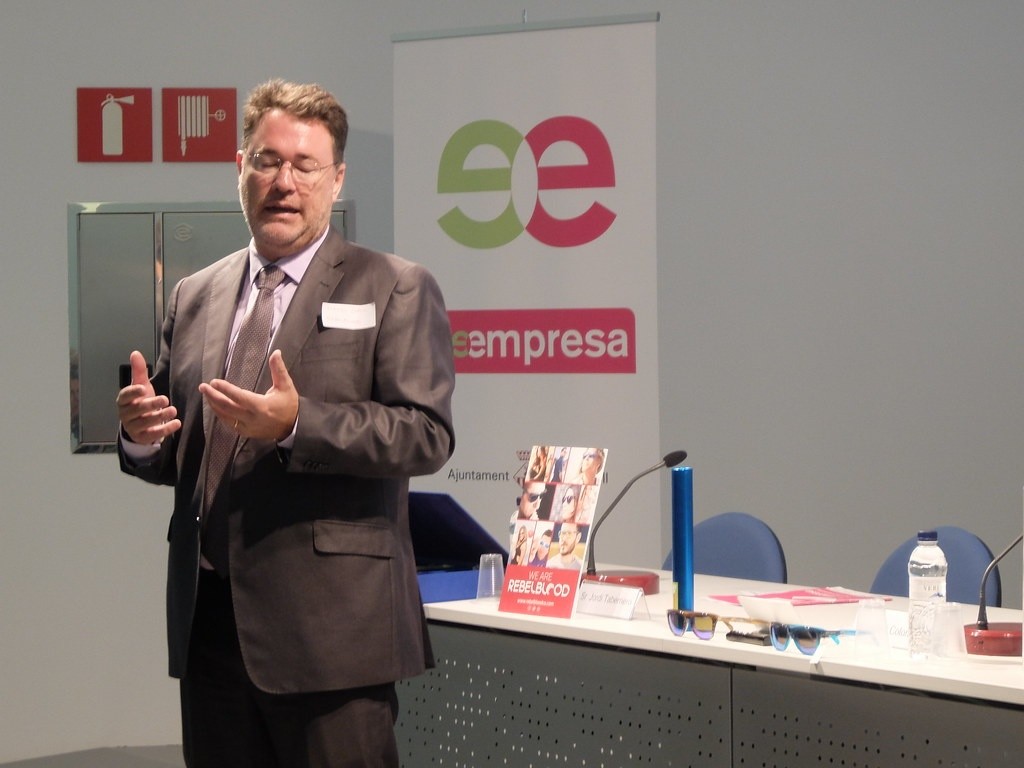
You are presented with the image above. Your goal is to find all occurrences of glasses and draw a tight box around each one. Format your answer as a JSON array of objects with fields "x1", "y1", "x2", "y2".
[
  {"x1": 769, "y1": 622, "x2": 855, "y2": 656},
  {"x1": 540, "y1": 541, "x2": 550, "y2": 548},
  {"x1": 583, "y1": 451, "x2": 600, "y2": 458},
  {"x1": 243, "y1": 145, "x2": 340, "y2": 184},
  {"x1": 668, "y1": 607, "x2": 732, "y2": 642},
  {"x1": 525, "y1": 489, "x2": 545, "y2": 502},
  {"x1": 562, "y1": 496, "x2": 575, "y2": 503},
  {"x1": 558, "y1": 528, "x2": 579, "y2": 538}
]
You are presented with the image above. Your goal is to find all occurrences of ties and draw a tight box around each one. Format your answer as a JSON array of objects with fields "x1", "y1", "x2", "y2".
[{"x1": 198, "y1": 263, "x2": 287, "y2": 572}]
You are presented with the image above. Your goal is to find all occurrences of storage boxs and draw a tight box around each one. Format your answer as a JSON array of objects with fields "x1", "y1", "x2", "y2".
[{"x1": 400, "y1": 490, "x2": 508, "y2": 604}]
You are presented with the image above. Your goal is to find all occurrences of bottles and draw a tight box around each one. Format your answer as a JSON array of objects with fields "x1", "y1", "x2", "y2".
[{"x1": 909, "y1": 530, "x2": 948, "y2": 652}]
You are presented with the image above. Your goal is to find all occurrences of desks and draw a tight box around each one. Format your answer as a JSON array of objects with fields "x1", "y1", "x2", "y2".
[{"x1": 386, "y1": 563, "x2": 1024, "y2": 768}]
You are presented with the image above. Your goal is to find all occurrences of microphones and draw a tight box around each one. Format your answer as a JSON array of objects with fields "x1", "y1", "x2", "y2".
[{"x1": 580, "y1": 451, "x2": 687, "y2": 595}]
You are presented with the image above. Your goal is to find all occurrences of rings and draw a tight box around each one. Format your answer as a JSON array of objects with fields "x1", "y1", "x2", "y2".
[{"x1": 233, "y1": 419, "x2": 239, "y2": 430}]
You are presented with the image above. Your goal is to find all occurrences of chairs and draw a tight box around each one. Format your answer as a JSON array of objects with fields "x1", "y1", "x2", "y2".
[
  {"x1": 662, "y1": 511, "x2": 786, "y2": 586},
  {"x1": 871, "y1": 524, "x2": 1001, "y2": 607}
]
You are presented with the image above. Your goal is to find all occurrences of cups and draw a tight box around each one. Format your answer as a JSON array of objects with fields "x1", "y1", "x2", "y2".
[{"x1": 477, "y1": 554, "x2": 504, "y2": 610}]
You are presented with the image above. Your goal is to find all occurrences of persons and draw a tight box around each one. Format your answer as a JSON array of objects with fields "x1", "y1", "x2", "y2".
[
  {"x1": 70, "y1": 364, "x2": 80, "y2": 437},
  {"x1": 117, "y1": 81, "x2": 456, "y2": 768},
  {"x1": 507, "y1": 446, "x2": 606, "y2": 569}
]
